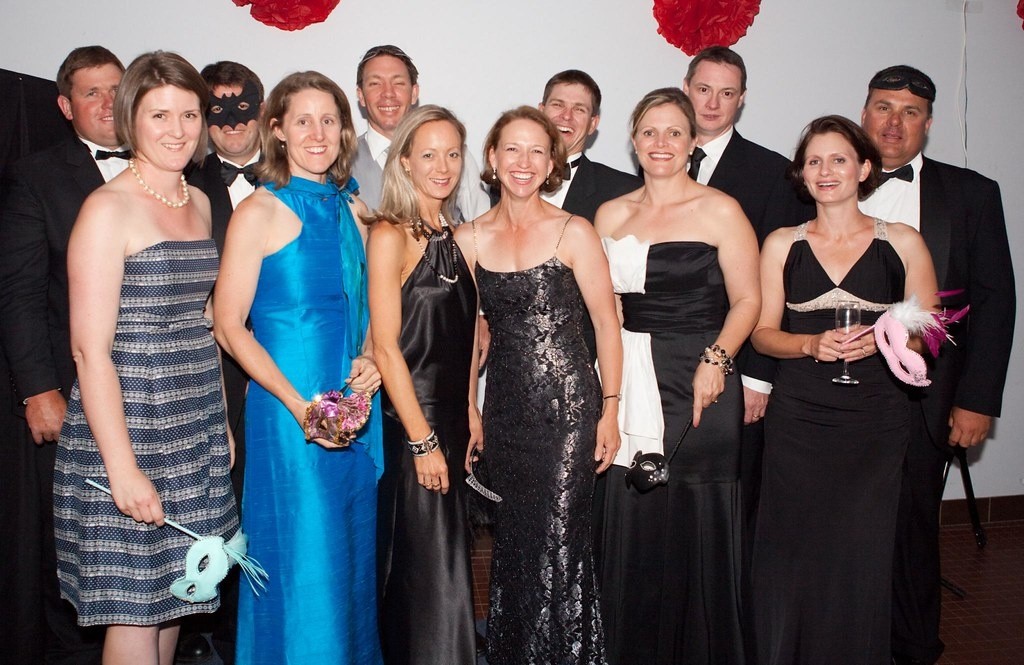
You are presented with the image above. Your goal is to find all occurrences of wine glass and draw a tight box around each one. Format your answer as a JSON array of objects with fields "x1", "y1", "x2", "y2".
[{"x1": 832, "y1": 300, "x2": 863, "y2": 385}]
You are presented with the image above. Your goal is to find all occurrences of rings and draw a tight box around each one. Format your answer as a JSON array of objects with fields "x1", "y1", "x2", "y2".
[
  {"x1": 433, "y1": 483, "x2": 440, "y2": 487},
  {"x1": 861, "y1": 347, "x2": 866, "y2": 356},
  {"x1": 711, "y1": 395, "x2": 718, "y2": 402},
  {"x1": 425, "y1": 483, "x2": 432, "y2": 487},
  {"x1": 371, "y1": 384, "x2": 375, "y2": 391},
  {"x1": 752, "y1": 414, "x2": 760, "y2": 418}
]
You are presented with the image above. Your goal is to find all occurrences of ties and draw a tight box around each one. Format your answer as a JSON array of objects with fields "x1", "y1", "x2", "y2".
[{"x1": 686, "y1": 147, "x2": 707, "y2": 183}]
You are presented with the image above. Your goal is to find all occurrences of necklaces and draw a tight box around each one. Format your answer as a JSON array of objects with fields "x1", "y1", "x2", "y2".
[
  {"x1": 410, "y1": 210, "x2": 459, "y2": 283},
  {"x1": 128, "y1": 158, "x2": 191, "y2": 208}
]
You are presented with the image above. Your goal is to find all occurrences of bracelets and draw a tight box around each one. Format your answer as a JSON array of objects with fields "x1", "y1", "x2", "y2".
[
  {"x1": 604, "y1": 394, "x2": 622, "y2": 401},
  {"x1": 406, "y1": 428, "x2": 439, "y2": 455},
  {"x1": 700, "y1": 345, "x2": 734, "y2": 375}
]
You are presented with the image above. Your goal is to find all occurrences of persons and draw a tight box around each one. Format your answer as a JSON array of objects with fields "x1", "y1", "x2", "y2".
[
  {"x1": 540, "y1": 72, "x2": 645, "y2": 227},
  {"x1": 369, "y1": 105, "x2": 490, "y2": 665},
  {"x1": 346, "y1": 42, "x2": 487, "y2": 224},
  {"x1": 858, "y1": 67, "x2": 1018, "y2": 665},
  {"x1": 752, "y1": 116, "x2": 940, "y2": 665},
  {"x1": 0, "y1": 45, "x2": 385, "y2": 665},
  {"x1": 592, "y1": 87, "x2": 762, "y2": 665},
  {"x1": 453, "y1": 105, "x2": 625, "y2": 665},
  {"x1": 685, "y1": 45, "x2": 816, "y2": 664}
]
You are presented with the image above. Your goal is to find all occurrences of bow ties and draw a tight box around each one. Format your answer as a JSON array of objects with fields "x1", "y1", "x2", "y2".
[
  {"x1": 876, "y1": 164, "x2": 914, "y2": 188},
  {"x1": 560, "y1": 163, "x2": 571, "y2": 181},
  {"x1": 220, "y1": 160, "x2": 263, "y2": 187},
  {"x1": 95, "y1": 149, "x2": 133, "y2": 161}
]
